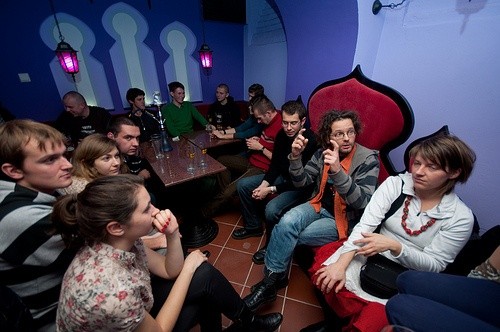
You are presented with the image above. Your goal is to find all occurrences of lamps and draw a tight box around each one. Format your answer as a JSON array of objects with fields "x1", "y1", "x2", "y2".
[
  {"x1": 49, "y1": 0, "x2": 79, "y2": 83},
  {"x1": 198, "y1": 0, "x2": 215, "y2": 82}
]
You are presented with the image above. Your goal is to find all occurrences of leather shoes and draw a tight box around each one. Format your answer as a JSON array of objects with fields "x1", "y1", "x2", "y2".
[
  {"x1": 252, "y1": 245, "x2": 267, "y2": 265},
  {"x1": 269, "y1": 293, "x2": 278, "y2": 302},
  {"x1": 231, "y1": 228, "x2": 264, "y2": 239},
  {"x1": 233, "y1": 312, "x2": 283, "y2": 332},
  {"x1": 202, "y1": 250, "x2": 210, "y2": 258},
  {"x1": 250, "y1": 270, "x2": 289, "y2": 293},
  {"x1": 232, "y1": 285, "x2": 269, "y2": 312}
]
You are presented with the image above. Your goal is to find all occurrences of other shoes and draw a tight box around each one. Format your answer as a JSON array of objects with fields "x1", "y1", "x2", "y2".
[{"x1": 300, "y1": 319, "x2": 337, "y2": 332}]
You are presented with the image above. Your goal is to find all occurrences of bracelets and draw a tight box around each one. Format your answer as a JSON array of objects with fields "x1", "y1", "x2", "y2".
[
  {"x1": 223, "y1": 130, "x2": 226, "y2": 135},
  {"x1": 271, "y1": 186, "x2": 277, "y2": 195},
  {"x1": 206, "y1": 124, "x2": 210, "y2": 131}
]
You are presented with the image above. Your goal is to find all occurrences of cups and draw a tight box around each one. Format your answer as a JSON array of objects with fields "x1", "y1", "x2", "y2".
[{"x1": 152, "y1": 138, "x2": 163, "y2": 159}]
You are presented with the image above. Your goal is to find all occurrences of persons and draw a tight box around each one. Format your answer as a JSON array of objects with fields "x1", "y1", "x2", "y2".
[
  {"x1": 300, "y1": 133, "x2": 477, "y2": 332},
  {"x1": 209, "y1": 83, "x2": 265, "y2": 156},
  {"x1": 55, "y1": 133, "x2": 210, "y2": 260},
  {"x1": 242, "y1": 108, "x2": 380, "y2": 312},
  {"x1": 160, "y1": 82, "x2": 213, "y2": 137},
  {"x1": 210, "y1": 93, "x2": 283, "y2": 215},
  {"x1": 126, "y1": 88, "x2": 161, "y2": 143},
  {"x1": 210, "y1": 83, "x2": 241, "y2": 131},
  {"x1": 52, "y1": 91, "x2": 114, "y2": 149},
  {"x1": 232, "y1": 100, "x2": 318, "y2": 265},
  {"x1": 0, "y1": 120, "x2": 167, "y2": 332},
  {"x1": 52, "y1": 174, "x2": 283, "y2": 332},
  {"x1": 385, "y1": 246, "x2": 500, "y2": 332},
  {"x1": 104, "y1": 116, "x2": 156, "y2": 206}
]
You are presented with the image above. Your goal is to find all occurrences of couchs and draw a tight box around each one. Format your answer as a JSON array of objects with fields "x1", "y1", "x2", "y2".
[{"x1": 145, "y1": 65, "x2": 449, "y2": 318}]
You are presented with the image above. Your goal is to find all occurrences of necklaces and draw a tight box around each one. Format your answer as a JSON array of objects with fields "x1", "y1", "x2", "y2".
[{"x1": 401, "y1": 195, "x2": 436, "y2": 236}]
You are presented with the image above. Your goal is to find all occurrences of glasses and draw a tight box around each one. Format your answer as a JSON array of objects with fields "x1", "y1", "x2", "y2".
[
  {"x1": 282, "y1": 121, "x2": 301, "y2": 127},
  {"x1": 328, "y1": 130, "x2": 357, "y2": 139}
]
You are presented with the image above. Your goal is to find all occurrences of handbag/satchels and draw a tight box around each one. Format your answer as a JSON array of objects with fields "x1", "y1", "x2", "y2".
[{"x1": 360, "y1": 253, "x2": 410, "y2": 299}]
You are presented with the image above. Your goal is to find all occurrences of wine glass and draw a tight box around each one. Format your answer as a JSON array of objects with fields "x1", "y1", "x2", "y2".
[
  {"x1": 198, "y1": 144, "x2": 208, "y2": 169},
  {"x1": 206, "y1": 115, "x2": 224, "y2": 142},
  {"x1": 185, "y1": 146, "x2": 197, "y2": 171}
]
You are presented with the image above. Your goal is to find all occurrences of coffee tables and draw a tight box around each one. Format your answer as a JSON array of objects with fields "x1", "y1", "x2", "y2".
[
  {"x1": 141, "y1": 136, "x2": 227, "y2": 248},
  {"x1": 182, "y1": 130, "x2": 240, "y2": 202}
]
